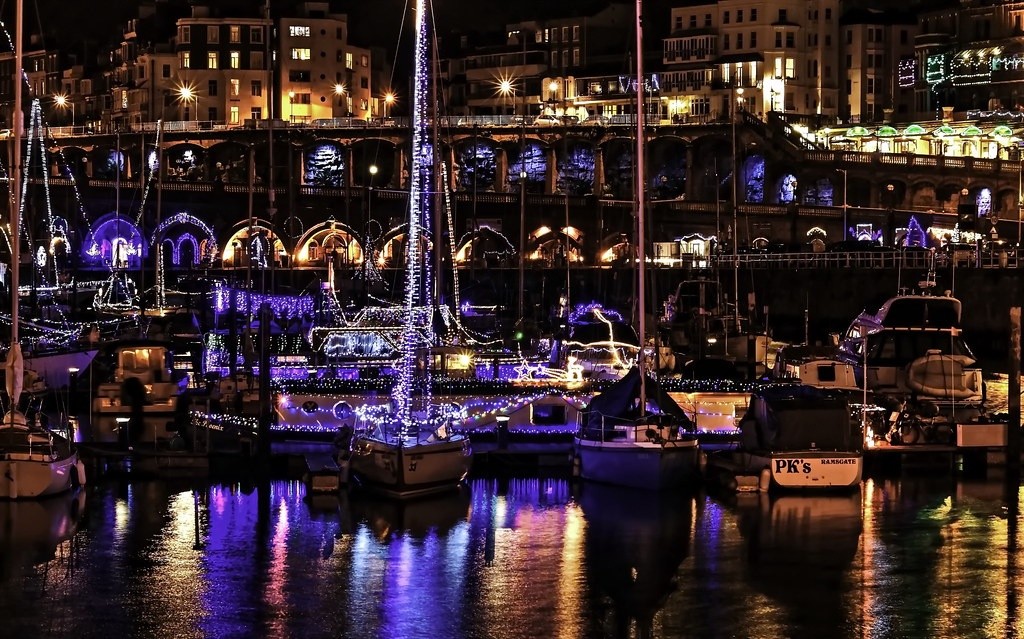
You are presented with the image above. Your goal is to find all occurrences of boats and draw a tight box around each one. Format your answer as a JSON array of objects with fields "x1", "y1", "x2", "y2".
[
  {"x1": 660, "y1": 270, "x2": 1012, "y2": 496},
  {"x1": 0, "y1": 0, "x2": 698, "y2": 499}
]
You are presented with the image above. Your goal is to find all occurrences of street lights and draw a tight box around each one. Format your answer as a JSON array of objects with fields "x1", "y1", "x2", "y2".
[
  {"x1": 56, "y1": 97, "x2": 75, "y2": 127},
  {"x1": 181, "y1": 88, "x2": 198, "y2": 121},
  {"x1": 770, "y1": 88, "x2": 775, "y2": 108},
  {"x1": 334, "y1": 85, "x2": 351, "y2": 128},
  {"x1": 385, "y1": 94, "x2": 402, "y2": 127},
  {"x1": 549, "y1": 82, "x2": 557, "y2": 118},
  {"x1": 289, "y1": 90, "x2": 295, "y2": 126},
  {"x1": 737, "y1": 87, "x2": 743, "y2": 109},
  {"x1": 501, "y1": 81, "x2": 516, "y2": 127}
]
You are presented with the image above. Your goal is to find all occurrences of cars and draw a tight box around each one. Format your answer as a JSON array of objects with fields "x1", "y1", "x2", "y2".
[
  {"x1": 581, "y1": 116, "x2": 610, "y2": 126},
  {"x1": 515, "y1": 115, "x2": 579, "y2": 126},
  {"x1": 0, "y1": 128, "x2": 15, "y2": 139}
]
